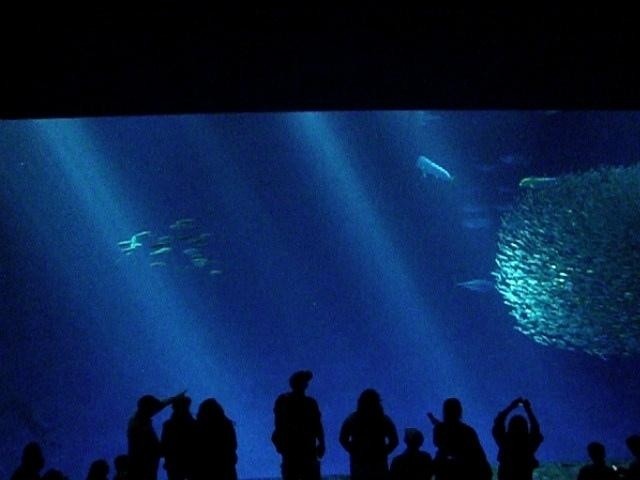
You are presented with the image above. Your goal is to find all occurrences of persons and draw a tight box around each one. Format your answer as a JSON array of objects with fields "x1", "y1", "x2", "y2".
[
  {"x1": 576, "y1": 441, "x2": 620, "y2": 480},
  {"x1": 271, "y1": 370, "x2": 326, "y2": 480},
  {"x1": 616, "y1": 434, "x2": 640, "y2": 480},
  {"x1": 9, "y1": 389, "x2": 238, "y2": 480},
  {"x1": 339, "y1": 388, "x2": 544, "y2": 480}
]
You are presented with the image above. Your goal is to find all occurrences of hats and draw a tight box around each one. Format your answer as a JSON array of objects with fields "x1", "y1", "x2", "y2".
[{"x1": 289, "y1": 370, "x2": 313, "y2": 388}]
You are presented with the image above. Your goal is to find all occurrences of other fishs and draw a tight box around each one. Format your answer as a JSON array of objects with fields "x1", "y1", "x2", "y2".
[
  {"x1": 456, "y1": 280, "x2": 495, "y2": 294},
  {"x1": 114, "y1": 218, "x2": 224, "y2": 278},
  {"x1": 414, "y1": 156, "x2": 454, "y2": 184},
  {"x1": 489, "y1": 159, "x2": 638, "y2": 362}
]
